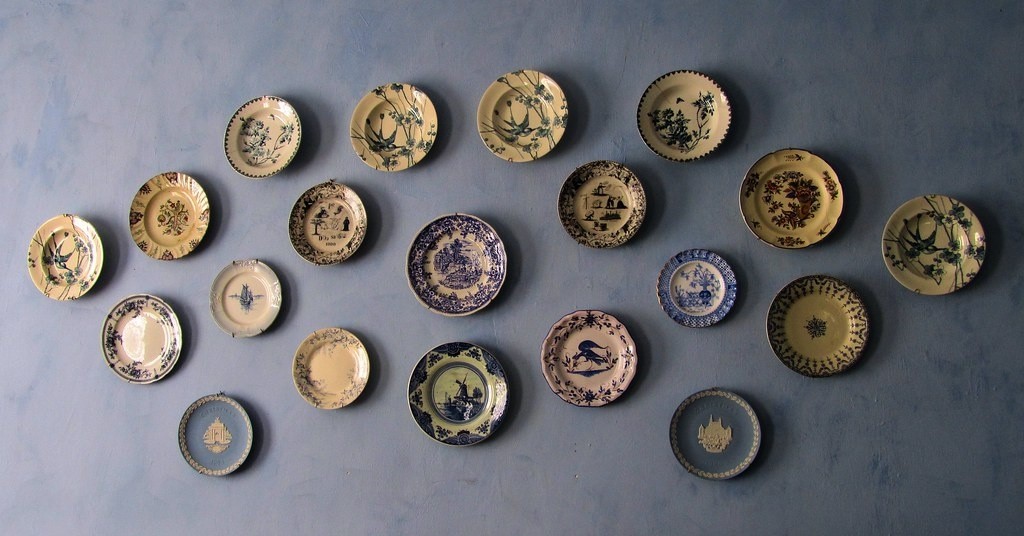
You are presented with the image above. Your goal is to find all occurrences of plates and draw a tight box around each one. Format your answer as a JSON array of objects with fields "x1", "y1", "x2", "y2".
[
  {"x1": 657, "y1": 249, "x2": 737, "y2": 327},
  {"x1": 556, "y1": 161, "x2": 646, "y2": 249},
  {"x1": 669, "y1": 389, "x2": 761, "y2": 481},
  {"x1": 407, "y1": 342, "x2": 510, "y2": 446},
  {"x1": 637, "y1": 70, "x2": 732, "y2": 163},
  {"x1": 541, "y1": 311, "x2": 638, "y2": 406},
  {"x1": 882, "y1": 195, "x2": 986, "y2": 296},
  {"x1": 209, "y1": 260, "x2": 282, "y2": 337},
  {"x1": 178, "y1": 395, "x2": 253, "y2": 476},
  {"x1": 350, "y1": 83, "x2": 438, "y2": 172},
  {"x1": 27, "y1": 215, "x2": 104, "y2": 299},
  {"x1": 405, "y1": 213, "x2": 506, "y2": 316},
  {"x1": 287, "y1": 182, "x2": 367, "y2": 265},
  {"x1": 130, "y1": 173, "x2": 210, "y2": 259},
  {"x1": 224, "y1": 96, "x2": 302, "y2": 180},
  {"x1": 100, "y1": 295, "x2": 182, "y2": 384},
  {"x1": 767, "y1": 275, "x2": 870, "y2": 378},
  {"x1": 739, "y1": 149, "x2": 845, "y2": 250},
  {"x1": 477, "y1": 70, "x2": 568, "y2": 162},
  {"x1": 292, "y1": 328, "x2": 370, "y2": 409}
]
